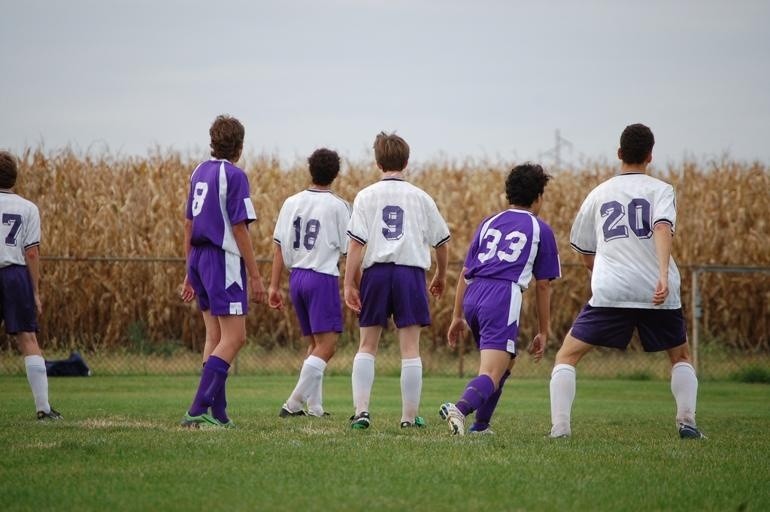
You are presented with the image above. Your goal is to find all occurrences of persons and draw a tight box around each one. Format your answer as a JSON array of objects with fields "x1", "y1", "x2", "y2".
[
  {"x1": 436, "y1": 162, "x2": 561, "y2": 432},
  {"x1": 268, "y1": 149, "x2": 360, "y2": 421},
  {"x1": 343, "y1": 131, "x2": 453, "y2": 427},
  {"x1": 0, "y1": 149, "x2": 66, "y2": 422},
  {"x1": 539, "y1": 122, "x2": 711, "y2": 442},
  {"x1": 181, "y1": 114, "x2": 268, "y2": 429}
]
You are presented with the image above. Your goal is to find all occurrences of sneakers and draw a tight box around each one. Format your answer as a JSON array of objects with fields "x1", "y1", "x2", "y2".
[
  {"x1": 401, "y1": 417, "x2": 425, "y2": 429},
  {"x1": 467, "y1": 423, "x2": 493, "y2": 433},
  {"x1": 37, "y1": 406, "x2": 64, "y2": 420},
  {"x1": 181, "y1": 411, "x2": 235, "y2": 427},
  {"x1": 439, "y1": 403, "x2": 465, "y2": 436},
  {"x1": 279, "y1": 408, "x2": 329, "y2": 416},
  {"x1": 679, "y1": 423, "x2": 704, "y2": 438},
  {"x1": 349, "y1": 412, "x2": 370, "y2": 429}
]
